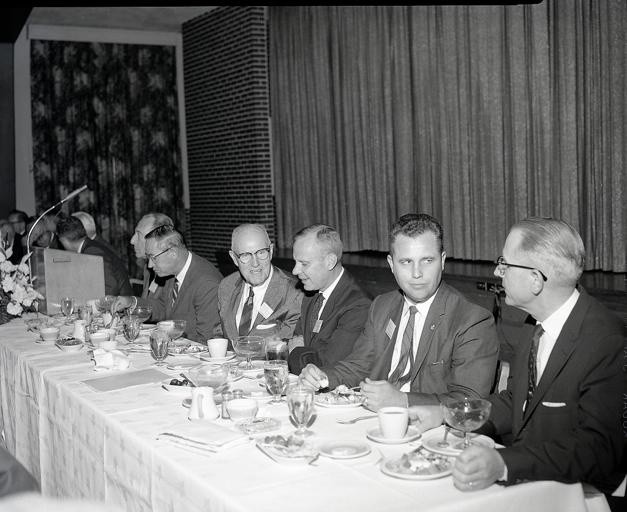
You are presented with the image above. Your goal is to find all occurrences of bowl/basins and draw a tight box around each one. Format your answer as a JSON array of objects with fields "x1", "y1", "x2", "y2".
[{"x1": 226, "y1": 399, "x2": 258, "y2": 421}]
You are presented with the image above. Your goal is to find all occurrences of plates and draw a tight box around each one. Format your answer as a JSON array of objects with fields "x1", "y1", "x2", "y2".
[
  {"x1": 35, "y1": 314, "x2": 269, "y2": 393},
  {"x1": 364, "y1": 424, "x2": 420, "y2": 445},
  {"x1": 318, "y1": 438, "x2": 372, "y2": 460},
  {"x1": 418, "y1": 429, "x2": 496, "y2": 456},
  {"x1": 314, "y1": 392, "x2": 362, "y2": 409},
  {"x1": 379, "y1": 449, "x2": 453, "y2": 480}
]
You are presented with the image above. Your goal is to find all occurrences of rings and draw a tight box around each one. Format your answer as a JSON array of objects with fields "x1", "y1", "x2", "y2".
[{"x1": 468, "y1": 482, "x2": 473, "y2": 489}]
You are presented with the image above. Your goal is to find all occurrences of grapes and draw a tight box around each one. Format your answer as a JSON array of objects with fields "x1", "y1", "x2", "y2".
[
  {"x1": 56, "y1": 336, "x2": 80, "y2": 346},
  {"x1": 170, "y1": 377, "x2": 189, "y2": 387}
]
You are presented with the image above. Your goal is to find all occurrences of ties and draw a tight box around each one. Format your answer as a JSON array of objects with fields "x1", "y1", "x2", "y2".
[
  {"x1": 170, "y1": 279, "x2": 178, "y2": 308},
  {"x1": 239, "y1": 286, "x2": 253, "y2": 336},
  {"x1": 310, "y1": 294, "x2": 325, "y2": 335},
  {"x1": 387, "y1": 306, "x2": 417, "y2": 388},
  {"x1": 528, "y1": 325, "x2": 544, "y2": 403}
]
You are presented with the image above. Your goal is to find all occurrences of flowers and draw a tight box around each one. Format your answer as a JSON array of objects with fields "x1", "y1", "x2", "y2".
[{"x1": 0, "y1": 231, "x2": 45, "y2": 324}]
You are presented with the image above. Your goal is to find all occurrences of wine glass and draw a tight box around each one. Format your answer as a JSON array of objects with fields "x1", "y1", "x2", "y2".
[
  {"x1": 60, "y1": 296, "x2": 153, "y2": 328},
  {"x1": 286, "y1": 384, "x2": 316, "y2": 439},
  {"x1": 440, "y1": 396, "x2": 493, "y2": 452},
  {"x1": 262, "y1": 359, "x2": 289, "y2": 406}
]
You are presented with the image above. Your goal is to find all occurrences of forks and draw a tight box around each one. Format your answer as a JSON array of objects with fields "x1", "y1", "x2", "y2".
[{"x1": 336, "y1": 413, "x2": 376, "y2": 427}]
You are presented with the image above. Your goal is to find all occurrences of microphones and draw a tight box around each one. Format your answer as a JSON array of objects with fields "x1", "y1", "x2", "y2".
[{"x1": 26, "y1": 184, "x2": 90, "y2": 313}]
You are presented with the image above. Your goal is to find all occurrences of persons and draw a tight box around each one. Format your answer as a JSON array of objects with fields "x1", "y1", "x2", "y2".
[
  {"x1": 0, "y1": 211, "x2": 225, "y2": 346},
  {"x1": 296, "y1": 214, "x2": 500, "y2": 413},
  {"x1": 216, "y1": 224, "x2": 304, "y2": 359},
  {"x1": 406, "y1": 216, "x2": 627, "y2": 512},
  {"x1": 278, "y1": 223, "x2": 373, "y2": 376}
]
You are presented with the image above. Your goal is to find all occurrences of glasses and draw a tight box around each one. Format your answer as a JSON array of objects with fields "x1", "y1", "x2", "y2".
[
  {"x1": 234, "y1": 248, "x2": 270, "y2": 263},
  {"x1": 497, "y1": 256, "x2": 548, "y2": 281},
  {"x1": 151, "y1": 248, "x2": 170, "y2": 263}
]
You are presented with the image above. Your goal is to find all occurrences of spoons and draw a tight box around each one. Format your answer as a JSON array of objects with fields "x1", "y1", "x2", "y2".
[{"x1": 437, "y1": 424, "x2": 450, "y2": 450}]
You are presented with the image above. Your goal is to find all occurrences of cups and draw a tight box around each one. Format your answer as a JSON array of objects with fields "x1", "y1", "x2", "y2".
[
  {"x1": 187, "y1": 386, "x2": 218, "y2": 424},
  {"x1": 375, "y1": 406, "x2": 408, "y2": 437}
]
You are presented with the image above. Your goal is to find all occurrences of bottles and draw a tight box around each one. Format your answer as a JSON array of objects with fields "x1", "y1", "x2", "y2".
[{"x1": 220, "y1": 390, "x2": 243, "y2": 418}]
